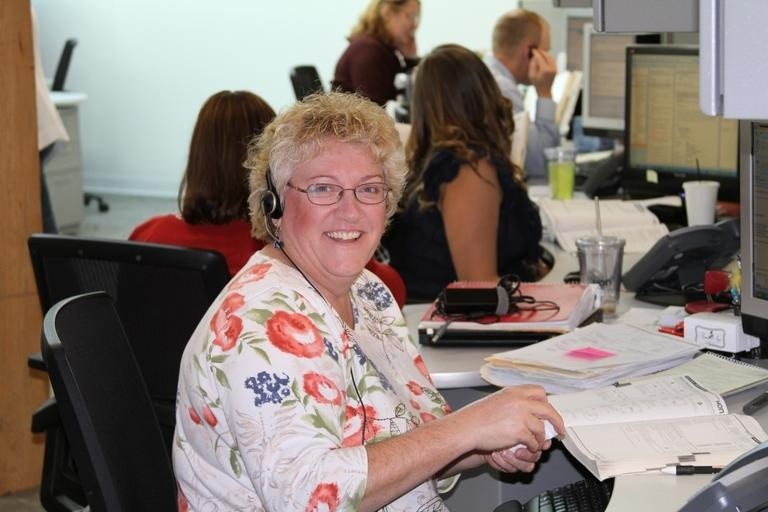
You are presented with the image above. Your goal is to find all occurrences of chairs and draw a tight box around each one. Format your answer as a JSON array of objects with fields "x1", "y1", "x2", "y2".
[
  {"x1": 18, "y1": 230, "x2": 234, "y2": 512},
  {"x1": 37, "y1": 292, "x2": 177, "y2": 511},
  {"x1": 51, "y1": 37, "x2": 110, "y2": 214},
  {"x1": 290, "y1": 64, "x2": 323, "y2": 101}
]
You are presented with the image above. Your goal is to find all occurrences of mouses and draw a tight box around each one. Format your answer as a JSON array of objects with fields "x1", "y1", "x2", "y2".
[{"x1": 565, "y1": 271, "x2": 584, "y2": 285}]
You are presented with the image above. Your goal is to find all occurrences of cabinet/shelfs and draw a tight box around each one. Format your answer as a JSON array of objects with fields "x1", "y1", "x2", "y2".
[{"x1": 42, "y1": 91, "x2": 85, "y2": 237}]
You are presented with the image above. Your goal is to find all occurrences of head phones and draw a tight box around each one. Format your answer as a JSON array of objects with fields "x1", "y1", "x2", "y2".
[{"x1": 257, "y1": 166, "x2": 281, "y2": 220}]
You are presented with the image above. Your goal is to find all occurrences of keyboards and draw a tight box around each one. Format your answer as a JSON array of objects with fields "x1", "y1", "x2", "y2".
[{"x1": 523, "y1": 478, "x2": 614, "y2": 512}]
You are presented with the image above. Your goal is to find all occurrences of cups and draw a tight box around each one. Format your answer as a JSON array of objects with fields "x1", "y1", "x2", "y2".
[
  {"x1": 574, "y1": 235, "x2": 626, "y2": 316},
  {"x1": 542, "y1": 147, "x2": 578, "y2": 201},
  {"x1": 682, "y1": 180, "x2": 720, "y2": 229}
]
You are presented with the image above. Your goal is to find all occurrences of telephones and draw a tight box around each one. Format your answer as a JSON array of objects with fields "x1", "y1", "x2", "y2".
[
  {"x1": 622, "y1": 218, "x2": 740, "y2": 305},
  {"x1": 678, "y1": 441, "x2": 768, "y2": 512},
  {"x1": 585, "y1": 153, "x2": 624, "y2": 198}
]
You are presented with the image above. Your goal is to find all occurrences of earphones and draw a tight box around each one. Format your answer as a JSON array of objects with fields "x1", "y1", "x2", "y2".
[{"x1": 529, "y1": 46, "x2": 538, "y2": 56}]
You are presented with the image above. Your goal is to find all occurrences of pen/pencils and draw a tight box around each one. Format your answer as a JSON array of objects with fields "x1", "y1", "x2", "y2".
[
  {"x1": 744, "y1": 390, "x2": 768, "y2": 415},
  {"x1": 658, "y1": 327, "x2": 684, "y2": 337}
]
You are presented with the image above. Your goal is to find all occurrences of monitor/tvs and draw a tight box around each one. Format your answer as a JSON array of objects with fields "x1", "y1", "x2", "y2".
[
  {"x1": 622, "y1": 46, "x2": 741, "y2": 197},
  {"x1": 581, "y1": 24, "x2": 702, "y2": 137},
  {"x1": 563, "y1": 7, "x2": 595, "y2": 73},
  {"x1": 740, "y1": 118, "x2": 768, "y2": 320}
]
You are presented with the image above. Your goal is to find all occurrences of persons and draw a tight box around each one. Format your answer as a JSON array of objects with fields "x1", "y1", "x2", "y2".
[
  {"x1": 329, "y1": 1, "x2": 422, "y2": 108},
  {"x1": 380, "y1": 43, "x2": 550, "y2": 307},
  {"x1": 482, "y1": 8, "x2": 563, "y2": 180},
  {"x1": 126, "y1": 89, "x2": 406, "y2": 309},
  {"x1": 171, "y1": 85, "x2": 567, "y2": 512}
]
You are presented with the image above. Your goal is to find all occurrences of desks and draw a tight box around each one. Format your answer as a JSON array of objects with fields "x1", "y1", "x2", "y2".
[{"x1": 394, "y1": 185, "x2": 768, "y2": 510}]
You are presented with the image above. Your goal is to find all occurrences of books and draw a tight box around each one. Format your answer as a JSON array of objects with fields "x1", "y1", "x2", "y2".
[
  {"x1": 541, "y1": 199, "x2": 671, "y2": 253},
  {"x1": 651, "y1": 351, "x2": 767, "y2": 399},
  {"x1": 547, "y1": 373, "x2": 768, "y2": 482},
  {"x1": 479, "y1": 321, "x2": 704, "y2": 396}
]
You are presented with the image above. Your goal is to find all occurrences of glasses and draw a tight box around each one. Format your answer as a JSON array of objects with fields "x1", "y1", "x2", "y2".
[{"x1": 286, "y1": 181, "x2": 394, "y2": 205}]
